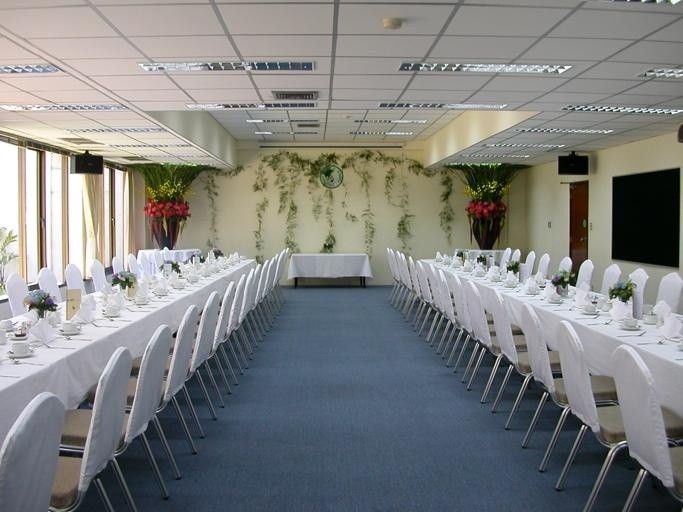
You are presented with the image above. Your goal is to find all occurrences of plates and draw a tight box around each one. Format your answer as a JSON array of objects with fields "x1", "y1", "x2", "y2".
[
  {"x1": 463, "y1": 270, "x2": 641, "y2": 329},
  {"x1": 0, "y1": 286, "x2": 184, "y2": 358}
]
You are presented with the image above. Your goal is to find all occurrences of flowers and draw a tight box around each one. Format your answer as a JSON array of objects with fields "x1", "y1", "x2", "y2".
[
  {"x1": 443, "y1": 163, "x2": 533, "y2": 221},
  {"x1": 128, "y1": 162, "x2": 221, "y2": 240}
]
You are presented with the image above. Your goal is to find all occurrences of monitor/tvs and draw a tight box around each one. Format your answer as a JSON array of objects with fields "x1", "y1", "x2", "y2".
[
  {"x1": 610, "y1": 168, "x2": 683, "y2": 269},
  {"x1": 557, "y1": 156, "x2": 591, "y2": 176}
]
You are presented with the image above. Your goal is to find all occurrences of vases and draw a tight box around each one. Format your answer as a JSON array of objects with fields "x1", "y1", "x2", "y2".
[
  {"x1": 472, "y1": 216, "x2": 500, "y2": 249},
  {"x1": 147, "y1": 217, "x2": 181, "y2": 250}
]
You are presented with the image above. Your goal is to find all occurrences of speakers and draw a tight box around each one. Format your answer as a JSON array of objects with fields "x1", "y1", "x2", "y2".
[{"x1": 70, "y1": 151, "x2": 102, "y2": 174}]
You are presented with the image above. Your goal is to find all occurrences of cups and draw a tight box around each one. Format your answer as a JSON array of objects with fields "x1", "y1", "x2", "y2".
[
  {"x1": 436, "y1": 257, "x2": 656, "y2": 327},
  {"x1": 0, "y1": 264, "x2": 240, "y2": 354}
]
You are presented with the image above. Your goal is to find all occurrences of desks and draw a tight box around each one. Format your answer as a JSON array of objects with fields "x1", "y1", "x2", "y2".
[{"x1": 287, "y1": 253, "x2": 375, "y2": 288}]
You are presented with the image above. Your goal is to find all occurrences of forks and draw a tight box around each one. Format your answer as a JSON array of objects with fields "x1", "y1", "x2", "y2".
[
  {"x1": 616, "y1": 331, "x2": 647, "y2": 337},
  {"x1": 637, "y1": 339, "x2": 666, "y2": 345}
]
